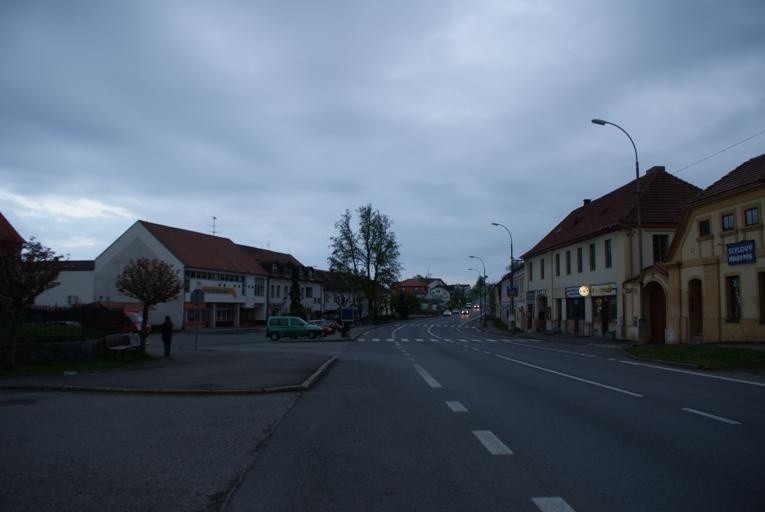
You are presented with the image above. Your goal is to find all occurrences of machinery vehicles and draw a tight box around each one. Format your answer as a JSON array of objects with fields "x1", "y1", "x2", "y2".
[{"x1": 75, "y1": 299, "x2": 157, "y2": 337}]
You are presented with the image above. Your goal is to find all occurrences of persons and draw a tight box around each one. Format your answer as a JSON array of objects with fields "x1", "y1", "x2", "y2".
[{"x1": 160, "y1": 315, "x2": 174, "y2": 358}]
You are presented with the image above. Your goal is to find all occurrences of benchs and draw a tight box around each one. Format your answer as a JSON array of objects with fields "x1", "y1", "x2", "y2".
[{"x1": 108, "y1": 344, "x2": 142, "y2": 360}]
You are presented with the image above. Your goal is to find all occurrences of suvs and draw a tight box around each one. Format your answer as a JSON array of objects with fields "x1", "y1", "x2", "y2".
[{"x1": 265, "y1": 317, "x2": 321, "y2": 340}]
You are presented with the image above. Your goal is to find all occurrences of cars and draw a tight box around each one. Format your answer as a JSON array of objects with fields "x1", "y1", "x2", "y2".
[
  {"x1": 442, "y1": 303, "x2": 480, "y2": 315},
  {"x1": 308, "y1": 319, "x2": 342, "y2": 336}
]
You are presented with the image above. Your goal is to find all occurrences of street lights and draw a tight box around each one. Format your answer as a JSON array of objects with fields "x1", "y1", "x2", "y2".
[
  {"x1": 591, "y1": 119, "x2": 648, "y2": 346},
  {"x1": 467, "y1": 255, "x2": 487, "y2": 325},
  {"x1": 491, "y1": 222, "x2": 515, "y2": 335}
]
